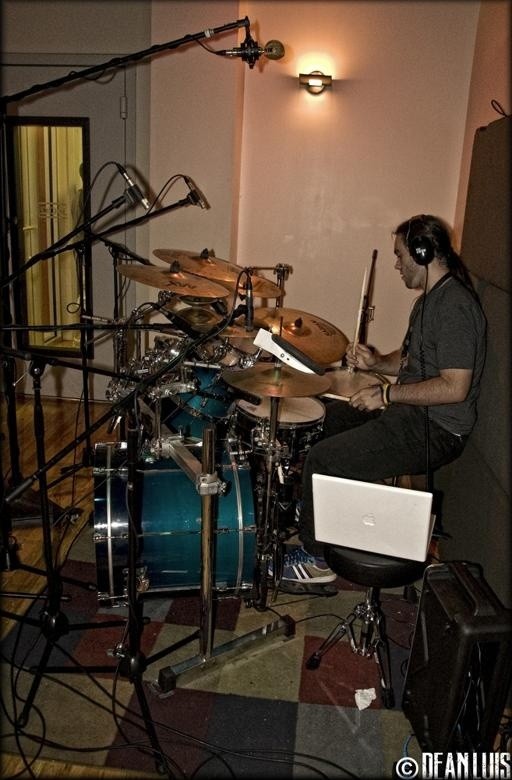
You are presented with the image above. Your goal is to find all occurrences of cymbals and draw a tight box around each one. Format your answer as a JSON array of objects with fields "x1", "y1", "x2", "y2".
[
  {"x1": 238, "y1": 307, "x2": 350, "y2": 364},
  {"x1": 178, "y1": 306, "x2": 223, "y2": 325},
  {"x1": 225, "y1": 275, "x2": 286, "y2": 299},
  {"x1": 223, "y1": 359, "x2": 333, "y2": 398},
  {"x1": 153, "y1": 247, "x2": 243, "y2": 283},
  {"x1": 117, "y1": 260, "x2": 231, "y2": 298}
]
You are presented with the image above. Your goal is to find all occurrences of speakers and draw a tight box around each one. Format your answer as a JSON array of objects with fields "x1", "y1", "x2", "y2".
[{"x1": 398, "y1": 561, "x2": 510, "y2": 778}]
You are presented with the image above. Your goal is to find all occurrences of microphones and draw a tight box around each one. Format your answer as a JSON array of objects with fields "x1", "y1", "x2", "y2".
[
  {"x1": 182, "y1": 171, "x2": 211, "y2": 213},
  {"x1": 115, "y1": 164, "x2": 150, "y2": 210},
  {"x1": 243, "y1": 277, "x2": 255, "y2": 333}
]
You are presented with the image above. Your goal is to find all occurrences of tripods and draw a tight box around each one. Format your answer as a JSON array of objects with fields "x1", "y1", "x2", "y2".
[{"x1": 0, "y1": 263, "x2": 227, "y2": 780}]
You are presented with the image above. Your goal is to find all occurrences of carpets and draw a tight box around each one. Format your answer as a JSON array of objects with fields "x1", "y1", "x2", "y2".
[{"x1": 3, "y1": 484, "x2": 425, "y2": 777}]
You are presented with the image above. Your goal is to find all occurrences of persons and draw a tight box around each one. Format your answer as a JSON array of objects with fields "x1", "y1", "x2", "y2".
[
  {"x1": 68, "y1": 159, "x2": 88, "y2": 297},
  {"x1": 262, "y1": 214, "x2": 489, "y2": 587}
]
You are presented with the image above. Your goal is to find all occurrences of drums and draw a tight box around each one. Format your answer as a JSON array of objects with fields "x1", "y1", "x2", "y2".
[
  {"x1": 235, "y1": 394, "x2": 327, "y2": 465},
  {"x1": 162, "y1": 363, "x2": 243, "y2": 438},
  {"x1": 89, "y1": 436, "x2": 257, "y2": 607}
]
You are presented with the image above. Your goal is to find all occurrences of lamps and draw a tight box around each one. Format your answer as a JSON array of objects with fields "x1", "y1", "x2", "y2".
[{"x1": 299, "y1": 71, "x2": 332, "y2": 95}]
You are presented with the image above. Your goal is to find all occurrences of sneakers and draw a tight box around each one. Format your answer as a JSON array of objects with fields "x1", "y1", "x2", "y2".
[{"x1": 268, "y1": 545, "x2": 337, "y2": 584}]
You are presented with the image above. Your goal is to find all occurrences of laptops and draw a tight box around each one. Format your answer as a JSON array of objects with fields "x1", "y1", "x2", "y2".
[{"x1": 310, "y1": 473, "x2": 436, "y2": 564}]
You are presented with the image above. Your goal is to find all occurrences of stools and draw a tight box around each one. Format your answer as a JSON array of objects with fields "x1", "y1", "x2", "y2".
[{"x1": 312, "y1": 543, "x2": 422, "y2": 708}]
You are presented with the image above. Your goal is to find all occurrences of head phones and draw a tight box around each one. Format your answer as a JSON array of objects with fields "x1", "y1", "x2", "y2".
[{"x1": 406, "y1": 213, "x2": 434, "y2": 265}]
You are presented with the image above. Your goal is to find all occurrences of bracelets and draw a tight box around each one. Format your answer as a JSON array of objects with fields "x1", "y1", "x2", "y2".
[{"x1": 380, "y1": 383, "x2": 392, "y2": 407}]
